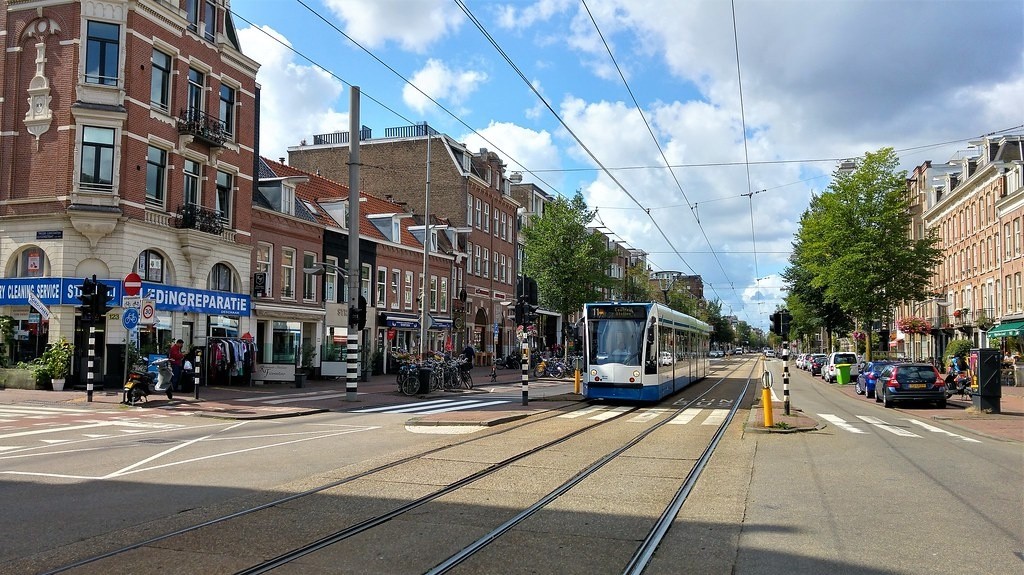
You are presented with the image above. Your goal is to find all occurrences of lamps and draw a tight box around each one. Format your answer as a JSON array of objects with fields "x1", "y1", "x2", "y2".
[
  {"x1": 509, "y1": 171, "x2": 528, "y2": 184},
  {"x1": 837, "y1": 157, "x2": 857, "y2": 171}
]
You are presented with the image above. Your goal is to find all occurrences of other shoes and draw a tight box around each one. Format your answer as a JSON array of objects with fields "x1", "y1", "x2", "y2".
[{"x1": 174, "y1": 389, "x2": 181, "y2": 392}]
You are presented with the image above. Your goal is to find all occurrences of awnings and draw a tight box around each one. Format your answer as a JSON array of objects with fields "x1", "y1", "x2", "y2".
[{"x1": 988, "y1": 322, "x2": 1024, "y2": 338}]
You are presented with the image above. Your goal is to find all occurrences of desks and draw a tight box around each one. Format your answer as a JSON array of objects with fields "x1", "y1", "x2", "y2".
[{"x1": 1000, "y1": 360, "x2": 1015, "y2": 385}]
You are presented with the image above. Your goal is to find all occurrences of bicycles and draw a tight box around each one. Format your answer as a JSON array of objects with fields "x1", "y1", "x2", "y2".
[
  {"x1": 489, "y1": 358, "x2": 502, "y2": 382},
  {"x1": 494, "y1": 352, "x2": 585, "y2": 379},
  {"x1": 397, "y1": 354, "x2": 476, "y2": 396}
]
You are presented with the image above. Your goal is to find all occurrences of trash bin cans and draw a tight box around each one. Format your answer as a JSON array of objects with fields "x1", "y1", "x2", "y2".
[
  {"x1": 419, "y1": 367, "x2": 433, "y2": 394},
  {"x1": 181, "y1": 369, "x2": 196, "y2": 393},
  {"x1": 836, "y1": 363, "x2": 852, "y2": 385}
]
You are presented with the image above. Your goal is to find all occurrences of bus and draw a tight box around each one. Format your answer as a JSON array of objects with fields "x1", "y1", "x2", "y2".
[{"x1": 575, "y1": 270, "x2": 711, "y2": 400}]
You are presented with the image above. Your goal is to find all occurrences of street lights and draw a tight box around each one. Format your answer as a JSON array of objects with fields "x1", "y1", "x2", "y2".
[{"x1": 910, "y1": 298, "x2": 946, "y2": 363}]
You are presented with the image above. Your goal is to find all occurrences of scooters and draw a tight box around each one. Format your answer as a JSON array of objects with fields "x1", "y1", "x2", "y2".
[
  {"x1": 943, "y1": 365, "x2": 972, "y2": 399},
  {"x1": 124, "y1": 358, "x2": 174, "y2": 404}
]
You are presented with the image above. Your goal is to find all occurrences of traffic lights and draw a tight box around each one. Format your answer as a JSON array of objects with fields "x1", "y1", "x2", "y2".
[
  {"x1": 98, "y1": 283, "x2": 117, "y2": 316},
  {"x1": 525, "y1": 304, "x2": 540, "y2": 324},
  {"x1": 82, "y1": 293, "x2": 97, "y2": 308},
  {"x1": 768, "y1": 313, "x2": 782, "y2": 335},
  {"x1": 351, "y1": 304, "x2": 366, "y2": 330},
  {"x1": 74, "y1": 278, "x2": 92, "y2": 313}
]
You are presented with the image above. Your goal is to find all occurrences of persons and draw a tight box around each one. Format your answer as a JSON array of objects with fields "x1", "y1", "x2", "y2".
[
  {"x1": 612, "y1": 342, "x2": 633, "y2": 363},
  {"x1": 170, "y1": 339, "x2": 187, "y2": 393},
  {"x1": 1001, "y1": 351, "x2": 1022, "y2": 377},
  {"x1": 541, "y1": 343, "x2": 567, "y2": 359},
  {"x1": 463, "y1": 343, "x2": 476, "y2": 372}
]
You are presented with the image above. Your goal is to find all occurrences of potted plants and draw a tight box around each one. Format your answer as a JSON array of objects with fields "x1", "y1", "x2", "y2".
[
  {"x1": 32, "y1": 336, "x2": 75, "y2": 391},
  {"x1": 293, "y1": 342, "x2": 318, "y2": 388}
]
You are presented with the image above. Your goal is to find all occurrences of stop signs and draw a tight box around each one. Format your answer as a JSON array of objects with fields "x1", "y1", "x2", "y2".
[{"x1": 123, "y1": 273, "x2": 142, "y2": 297}]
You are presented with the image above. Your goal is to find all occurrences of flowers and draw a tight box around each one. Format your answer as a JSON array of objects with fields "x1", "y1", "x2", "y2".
[
  {"x1": 896, "y1": 315, "x2": 932, "y2": 337},
  {"x1": 852, "y1": 331, "x2": 866, "y2": 340}
]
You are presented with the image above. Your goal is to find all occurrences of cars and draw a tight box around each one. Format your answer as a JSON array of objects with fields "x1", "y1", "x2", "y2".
[
  {"x1": 795, "y1": 352, "x2": 947, "y2": 409},
  {"x1": 766, "y1": 350, "x2": 775, "y2": 358},
  {"x1": 711, "y1": 347, "x2": 742, "y2": 357}
]
